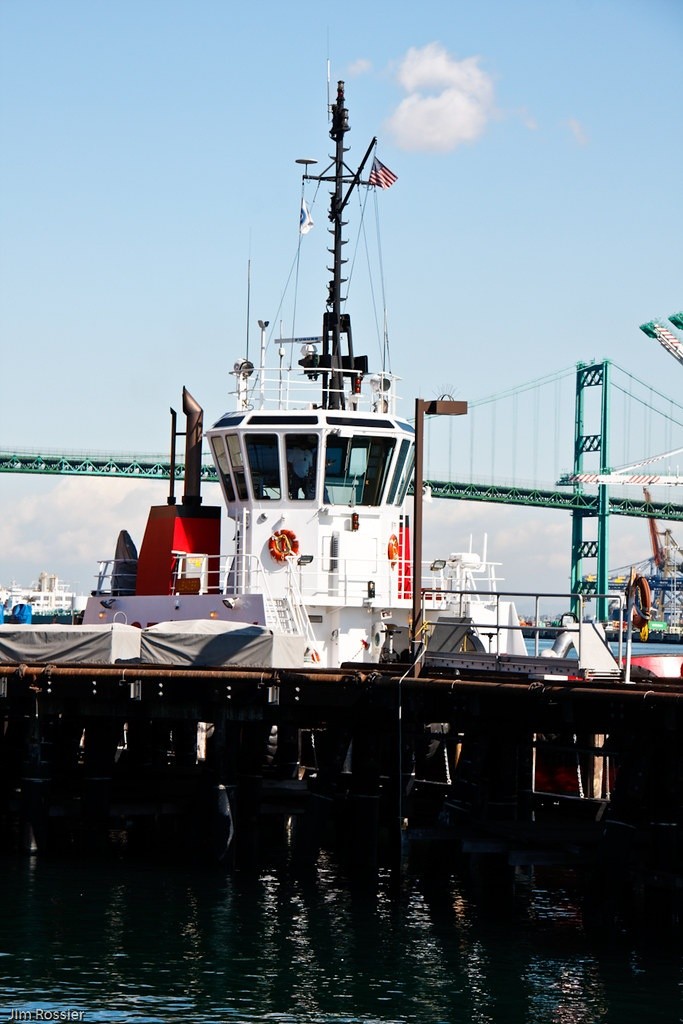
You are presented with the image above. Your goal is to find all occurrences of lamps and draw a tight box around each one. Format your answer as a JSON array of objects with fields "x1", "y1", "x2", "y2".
[
  {"x1": 222, "y1": 597, "x2": 244, "y2": 610},
  {"x1": 430, "y1": 560, "x2": 446, "y2": 572},
  {"x1": 99, "y1": 599, "x2": 116, "y2": 609},
  {"x1": 297, "y1": 554, "x2": 313, "y2": 566},
  {"x1": 234, "y1": 357, "x2": 254, "y2": 378},
  {"x1": 370, "y1": 374, "x2": 391, "y2": 391}
]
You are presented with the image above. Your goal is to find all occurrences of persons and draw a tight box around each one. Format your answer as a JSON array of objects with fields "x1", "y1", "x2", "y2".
[
  {"x1": 288, "y1": 437, "x2": 314, "y2": 500},
  {"x1": 0, "y1": 597, "x2": 8, "y2": 625}
]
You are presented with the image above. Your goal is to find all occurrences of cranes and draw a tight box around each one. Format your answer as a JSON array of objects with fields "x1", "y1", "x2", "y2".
[
  {"x1": 564, "y1": 487, "x2": 683, "y2": 632},
  {"x1": 553, "y1": 312, "x2": 683, "y2": 490}
]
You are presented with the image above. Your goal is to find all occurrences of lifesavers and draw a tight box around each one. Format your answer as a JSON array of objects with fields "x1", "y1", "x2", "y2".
[
  {"x1": 625, "y1": 573, "x2": 652, "y2": 628},
  {"x1": 388, "y1": 534, "x2": 399, "y2": 565},
  {"x1": 268, "y1": 529, "x2": 299, "y2": 562}
]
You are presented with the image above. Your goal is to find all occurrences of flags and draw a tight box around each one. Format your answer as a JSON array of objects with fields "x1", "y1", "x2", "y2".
[
  {"x1": 300, "y1": 198, "x2": 313, "y2": 234},
  {"x1": 368, "y1": 156, "x2": 398, "y2": 192}
]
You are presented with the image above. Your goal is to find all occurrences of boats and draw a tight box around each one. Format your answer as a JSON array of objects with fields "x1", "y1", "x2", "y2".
[{"x1": 81, "y1": 58, "x2": 530, "y2": 682}]
